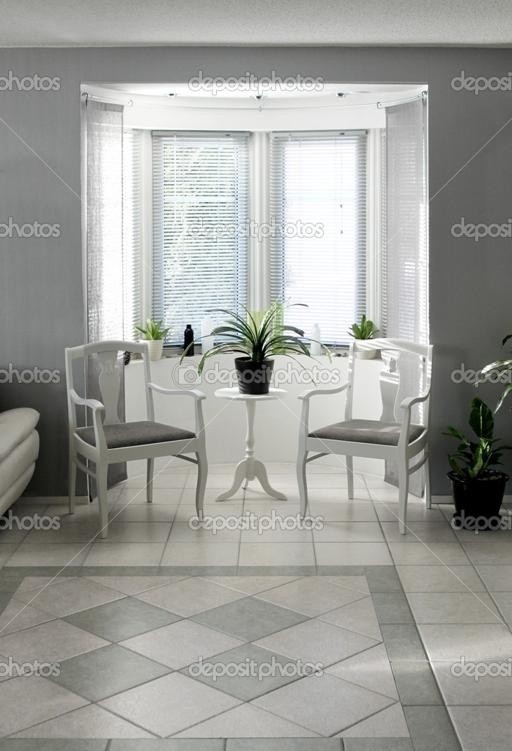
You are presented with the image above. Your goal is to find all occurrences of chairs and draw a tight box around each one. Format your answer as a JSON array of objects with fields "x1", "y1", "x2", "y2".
[
  {"x1": 295, "y1": 338, "x2": 434, "y2": 535},
  {"x1": 64, "y1": 338, "x2": 208, "y2": 539},
  {"x1": 0, "y1": 406, "x2": 40, "y2": 523}
]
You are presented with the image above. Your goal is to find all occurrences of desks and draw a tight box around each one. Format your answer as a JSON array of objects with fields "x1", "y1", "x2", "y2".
[{"x1": 214, "y1": 388, "x2": 288, "y2": 502}]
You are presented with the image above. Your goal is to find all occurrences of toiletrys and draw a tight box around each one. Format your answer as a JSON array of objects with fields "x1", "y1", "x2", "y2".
[
  {"x1": 310, "y1": 323, "x2": 323, "y2": 355},
  {"x1": 199, "y1": 320, "x2": 214, "y2": 355},
  {"x1": 184, "y1": 324, "x2": 194, "y2": 356}
]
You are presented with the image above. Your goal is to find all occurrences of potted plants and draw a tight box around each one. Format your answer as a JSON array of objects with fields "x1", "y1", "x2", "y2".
[
  {"x1": 179, "y1": 297, "x2": 333, "y2": 395},
  {"x1": 347, "y1": 314, "x2": 380, "y2": 360},
  {"x1": 135, "y1": 318, "x2": 173, "y2": 361},
  {"x1": 440, "y1": 399, "x2": 512, "y2": 532}
]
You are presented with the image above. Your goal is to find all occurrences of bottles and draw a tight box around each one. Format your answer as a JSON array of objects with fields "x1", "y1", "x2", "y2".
[
  {"x1": 310, "y1": 325, "x2": 322, "y2": 357},
  {"x1": 183, "y1": 324, "x2": 195, "y2": 357},
  {"x1": 200, "y1": 316, "x2": 214, "y2": 356}
]
